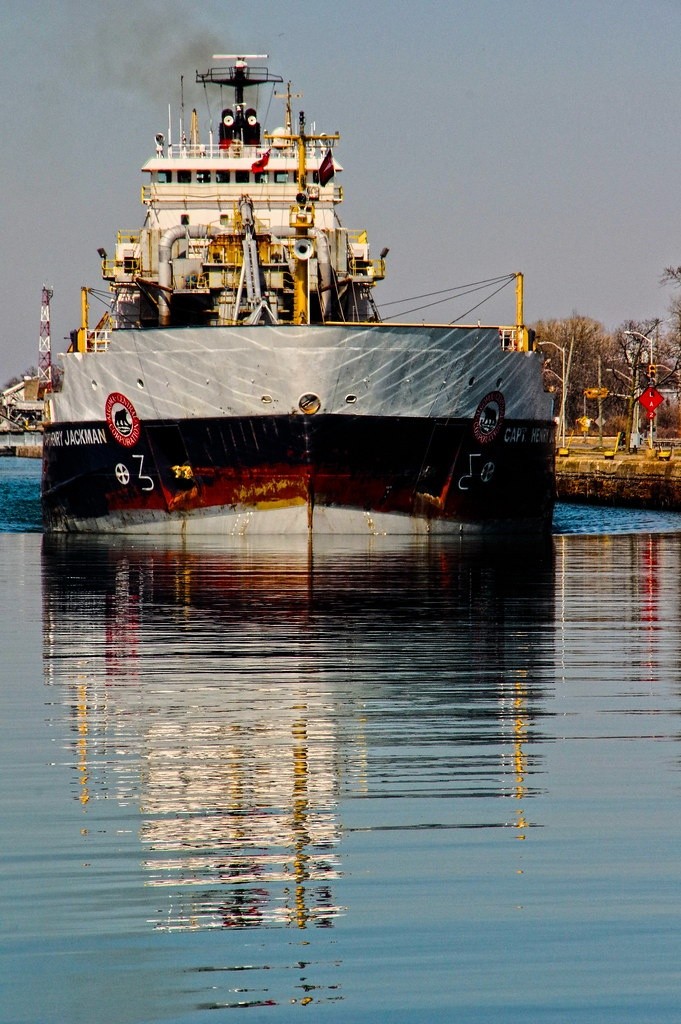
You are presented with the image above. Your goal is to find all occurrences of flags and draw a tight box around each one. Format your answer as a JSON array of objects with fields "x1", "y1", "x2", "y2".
[
  {"x1": 319, "y1": 149, "x2": 334, "y2": 185},
  {"x1": 253, "y1": 149, "x2": 271, "y2": 172}
]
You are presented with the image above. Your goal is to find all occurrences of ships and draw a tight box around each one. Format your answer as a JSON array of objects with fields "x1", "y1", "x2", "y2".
[{"x1": 37, "y1": 56, "x2": 561, "y2": 546}]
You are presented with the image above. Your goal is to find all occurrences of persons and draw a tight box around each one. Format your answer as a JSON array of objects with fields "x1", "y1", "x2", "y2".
[
  {"x1": 655, "y1": 442, "x2": 664, "y2": 456},
  {"x1": 668, "y1": 445, "x2": 676, "y2": 460},
  {"x1": 633, "y1": 445, "x2": 638, "y2": 454},
  {"x1": 19, "y1": 414, "x2": 35, "y2": 430}
]
